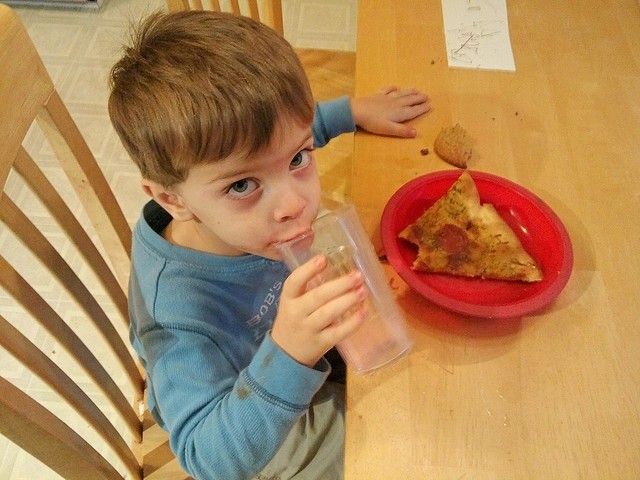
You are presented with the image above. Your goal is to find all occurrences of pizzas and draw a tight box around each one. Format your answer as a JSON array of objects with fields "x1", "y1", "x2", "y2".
[{"x1": 400, "y1": 169, "x2": 544, "y2": 285}]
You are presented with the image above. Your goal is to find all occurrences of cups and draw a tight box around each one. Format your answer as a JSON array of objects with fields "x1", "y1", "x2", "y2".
[{"x1": 276, "y1": 204, "x2": 412, "y2": 375}]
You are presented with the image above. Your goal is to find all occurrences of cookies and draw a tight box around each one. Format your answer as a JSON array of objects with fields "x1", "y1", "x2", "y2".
[{"x1": 434, "y1": 124, "x2": 476, "y2": 169}]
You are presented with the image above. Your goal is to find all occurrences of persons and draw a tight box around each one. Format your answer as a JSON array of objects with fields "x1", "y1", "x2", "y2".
[{"x1": 108, "y1": 10, "x2": 431, "y2": 478}]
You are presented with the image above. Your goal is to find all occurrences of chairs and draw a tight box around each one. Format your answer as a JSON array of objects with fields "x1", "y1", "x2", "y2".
[
  {"x1": 0, "y1": 2, "x2": 192, "y2": 480},
  {"x1": 167, "y1": 1, "x2": 357, "y2": 217}
]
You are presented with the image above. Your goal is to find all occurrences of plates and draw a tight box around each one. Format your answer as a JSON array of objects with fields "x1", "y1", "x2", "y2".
[{"x1": 381, "y1": 169, "x2": 573, "y2": 319}]
